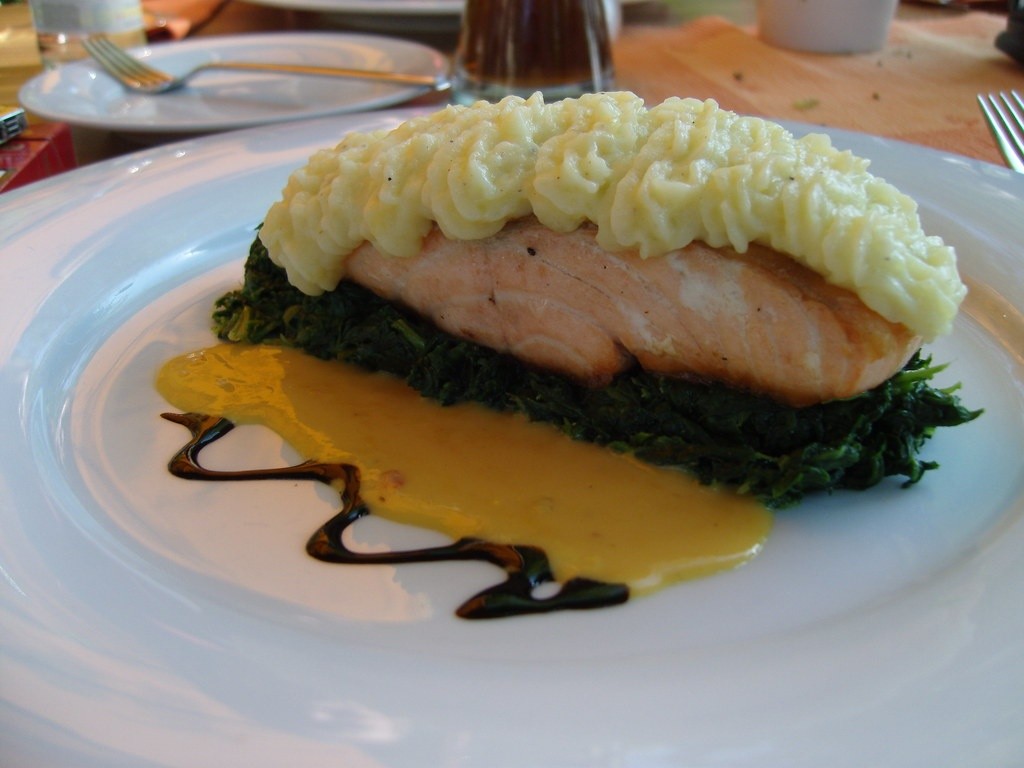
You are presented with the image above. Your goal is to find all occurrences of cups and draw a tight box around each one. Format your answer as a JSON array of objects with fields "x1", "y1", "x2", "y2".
[
  {"x1": 757, "y1": 0, "x2": 900, "y2": 52},
  {"x1": 30, "y1": 0, "x2": 145, "y2": 71},
  {"x1": 452, "y1": 0, "x2": 615, "y2": 102}
]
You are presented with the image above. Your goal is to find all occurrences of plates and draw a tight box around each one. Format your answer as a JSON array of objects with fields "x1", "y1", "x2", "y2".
[
  {"x1": 0, "y1": 104, "x2": 1024, "y2": 767},
  {"x1": 19, "y1": 31, "x2": 448, "y2": 130}
]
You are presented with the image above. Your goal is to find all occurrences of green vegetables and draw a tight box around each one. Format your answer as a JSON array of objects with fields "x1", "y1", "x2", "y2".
[{"x1": 216, "y1": 250, "x2": 981, "y2": 500}]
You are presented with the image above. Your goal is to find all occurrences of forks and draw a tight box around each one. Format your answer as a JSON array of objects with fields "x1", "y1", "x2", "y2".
[
  {"x1": 80, "y1": 34, "x2": 440, "y2": 92},
  {"x1": 976, "y1": 89, "x2": 1024, "y2": 176}
]
